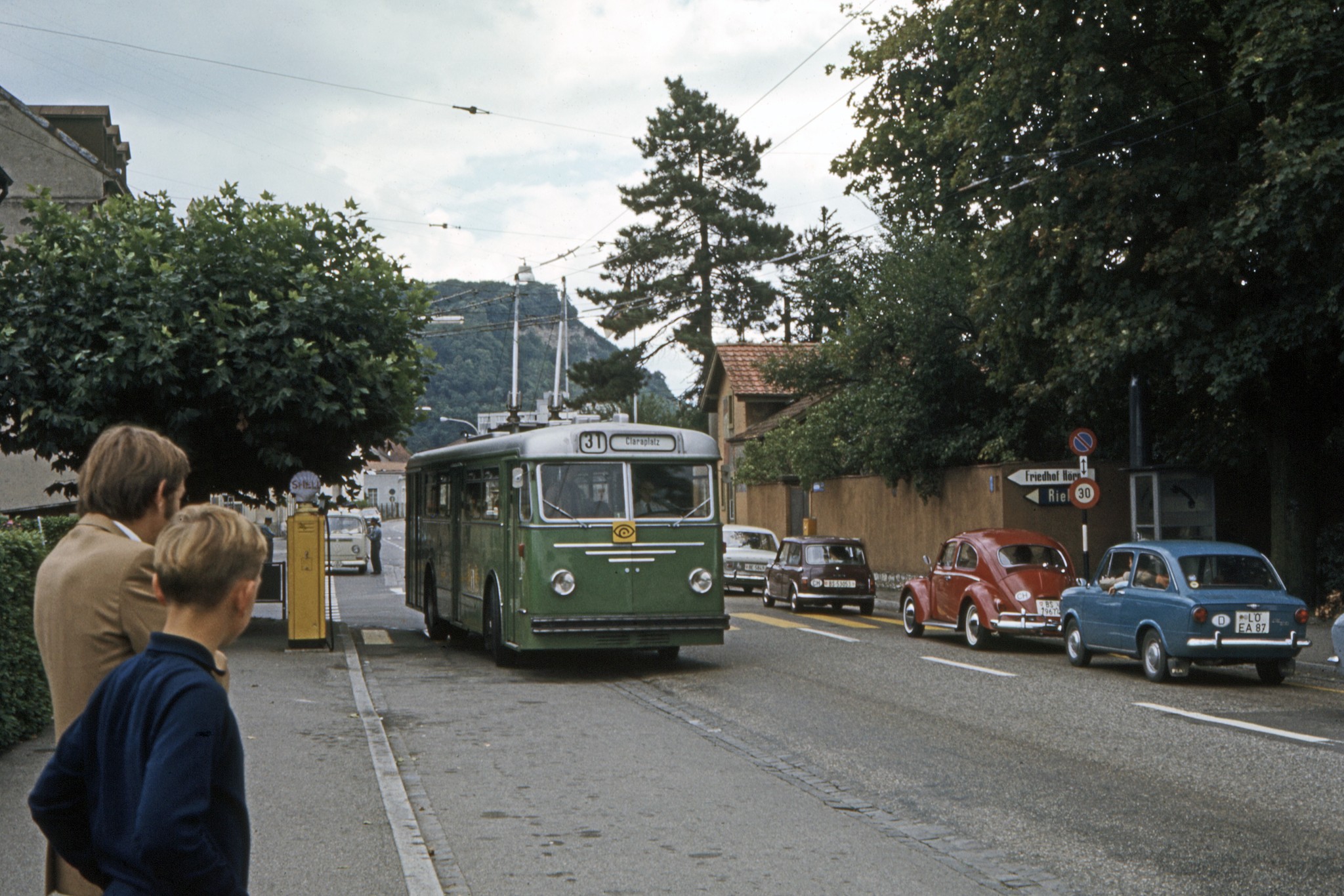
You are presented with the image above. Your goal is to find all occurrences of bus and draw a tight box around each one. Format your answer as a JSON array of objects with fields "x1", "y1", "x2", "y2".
[{"x1": 406, "y1": 422, "x2": 729, "y2": 671}]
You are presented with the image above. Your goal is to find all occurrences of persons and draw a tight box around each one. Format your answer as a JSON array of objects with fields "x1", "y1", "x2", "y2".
[
  {"x1": 364, "y1": 516, "x2": 382, "y2": 575},
  {"x1": 630, "y1": 481, "x2": 669, "y2": 517},
  {"x1": 1108, "y1": 553, "x2": 1151, "y2": 592},
  {"x1": 964, "y1": 547, "x2": 978, "y2": 568},
  {"x1": 1011, "y1": 546, "x2": 1035, "y2": 566},
  {"x1": 544, "y1": 465, "x2": 592, "y2": 520},
  {"x1": 260, "y1": 516, "x2": 276, "y2": 562},
  {"x1": 20, "y1": 423, "x2": 231, "y2": 895},
  {"x1": 588, "y1": 499, "x2": 610, "y2": 518},
  {"x1": 27, "y1": 503, "x2": 276, "y2": 896}
]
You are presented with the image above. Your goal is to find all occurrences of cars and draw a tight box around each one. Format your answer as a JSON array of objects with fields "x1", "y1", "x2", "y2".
[
  {"x1": 358, "y1": 507, "x2": 381, "y2": 526},
  {"x1": 899, "y1": 526, "x2": 1074, "y2": 649},
  {"x1": 1056, "y1": 537, "x2": 1313, "y2": 687},
  {"x1": 761, "y1": 535, "x2": 877, "y2": 616},
  {"x1": 321, "y1": 510, "x2": 368, "y2": 572},
  {"x1": 719, "y1": 523, "x2": 782, "y2": 593}
]
